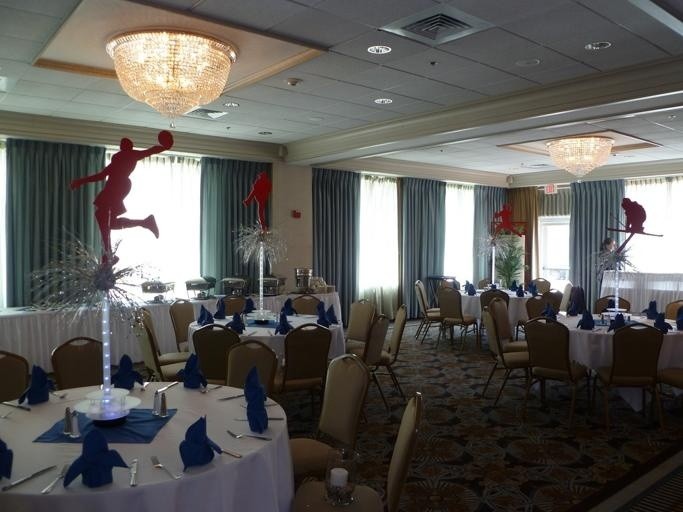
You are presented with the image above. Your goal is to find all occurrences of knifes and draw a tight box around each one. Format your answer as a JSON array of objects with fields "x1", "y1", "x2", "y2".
[
  {"x1": 154, "y1": 382, "x2": 179, "y2": 392},
  {"x1": 232, "y1": 417, "x2": 285, "y2": 422},
  {"x1": 215, "y1": 392, "x2": 246, "y2": 401},
  {"x1": 2, "y1": 465, "x2": 56, "y2": 492},
  {"x1": 127, "y1": 458, "x2": 138, "y2": 487},
  {"x1": 2, "y1": 401, "x2": 31, "y2": 412}
]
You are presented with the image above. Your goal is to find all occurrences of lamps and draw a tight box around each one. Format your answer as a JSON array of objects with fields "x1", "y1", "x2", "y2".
[
  {"x1": 544, "y1": 135, "x2": 615, "y2": 184},
  {"x1": 104, "y1": 29, "x2": 237, "y2": 130}
]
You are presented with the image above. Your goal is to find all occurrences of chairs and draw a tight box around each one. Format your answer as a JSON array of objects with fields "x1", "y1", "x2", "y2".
[{"x1": 1, "y1": 276, "x2": 683, "y2": 512}]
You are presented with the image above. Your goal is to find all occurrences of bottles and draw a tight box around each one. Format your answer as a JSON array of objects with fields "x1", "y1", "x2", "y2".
[
  {"x1": 625, "y1": 316, "x2": 630, "y2": 324},
  {"x1": 63, "y1": 405, "x2": 82, "y2": 439},
  {"x1": 239, "y1": 312, "x2": 248, "y2": 327},
  {"x1": 598, "y1": 314, "x2": 604, "y2": 325},
  {"x1": 152, "y1": 389, "x2": 167, "y2": 419},
  {"x1": 605, "y1": 314, "x2": 611, "y2": 326},
  {"x1": 274, "y1": 311, "x2": 279, "y2": 323}
]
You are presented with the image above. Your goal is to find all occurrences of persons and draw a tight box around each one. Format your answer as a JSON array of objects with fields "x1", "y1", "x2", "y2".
[
  {"x1": 618, "y1": 197, "x2": 646, "y2": 232},
  {"x1": 70, "y1": 130, "x2": 174, "y2": 264},
  {"x1": 599, "y1": 238, "x2": 621, "y2": 298},
  {"x1": 243, "y1": 171, "x2": 272, "y2": 231},
  {"x1": 492, "y1": 204, "x2": 523, "y2": 237}
]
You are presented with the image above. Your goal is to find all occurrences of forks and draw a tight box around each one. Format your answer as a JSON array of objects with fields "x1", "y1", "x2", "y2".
[
  {"x1": 197, "y1": 385, "x2": 223, "y2": 395},
  {"x1": 149, "y1": 454, "x2": 180, "y2": 480},
  {"x1": 225, "y1": 426, "x2": 275, "y2": 442},
  {"x1": 49, "y1": 390, "x2": 69, "y2": 399},
  {"x1": 40, "y1": 465, "x2": 70, "y2": 494}
]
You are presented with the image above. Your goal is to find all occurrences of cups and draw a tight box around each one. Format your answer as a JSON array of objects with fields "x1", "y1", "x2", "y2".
[
  {"x1": 326, "y1": 286, "x2": 335, "y2": 293},
  {"x1": 320, "y1": 446, "x2": 361, "y2": 508},
  {"x1": 318, "y1": 286, "x2": 326, "y2": 294}
]
